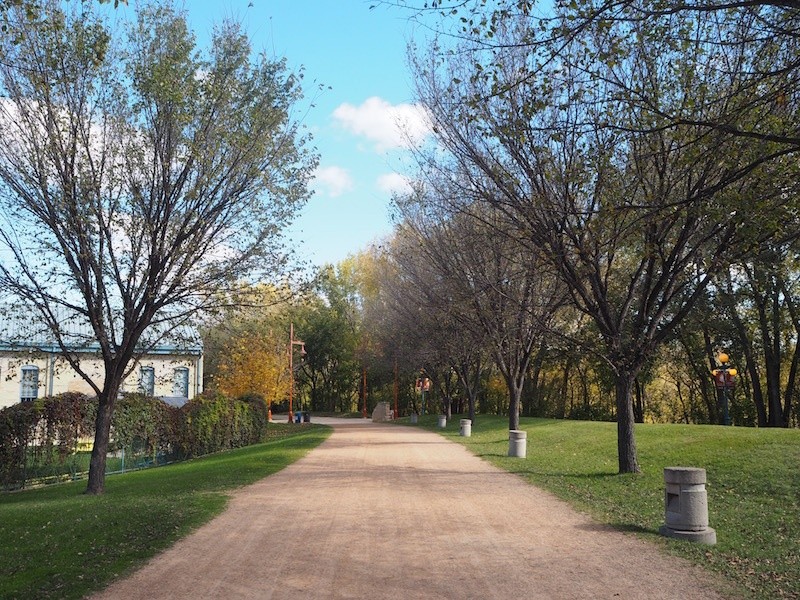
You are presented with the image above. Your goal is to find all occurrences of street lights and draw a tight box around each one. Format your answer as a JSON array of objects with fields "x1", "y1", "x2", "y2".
[
  {"x1": 712, "y1": 353, "x2": 737, "y2": 426},
  {"x1": 287, "y1": 323, "x2": 307, "y2": 424}
]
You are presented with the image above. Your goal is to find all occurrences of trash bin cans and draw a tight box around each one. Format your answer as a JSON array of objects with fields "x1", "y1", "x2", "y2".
[
  {"x1": 302, "y1": 412, "x2": 310, "y2": 422},
  {"x1": 295, "y1": 412, "x2": 302, "y2": 423}
]
